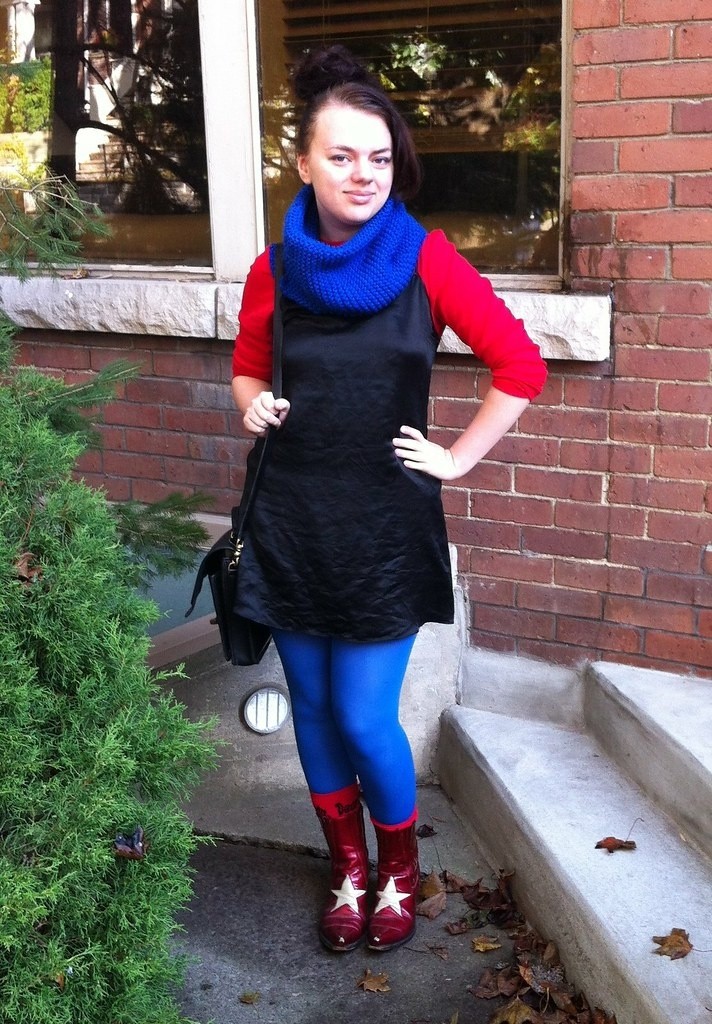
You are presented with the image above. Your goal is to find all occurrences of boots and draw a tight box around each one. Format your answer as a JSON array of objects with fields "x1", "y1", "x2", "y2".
[
  {"x1": 315, "y1": 804, "x2": 371, "y2": 952},
  {"x1": 366, "y1": 819, "x2": 420, "y2": 953}
]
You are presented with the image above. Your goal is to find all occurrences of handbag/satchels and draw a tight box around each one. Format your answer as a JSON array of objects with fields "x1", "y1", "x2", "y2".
[{"x1": 183, "y1": 528, "x2": 274, "y2": 668}]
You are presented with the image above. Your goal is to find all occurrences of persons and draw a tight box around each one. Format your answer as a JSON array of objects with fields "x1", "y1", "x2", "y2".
[{"x1": 229, "y1": 43, "x2": 551, "y2": 955}]
